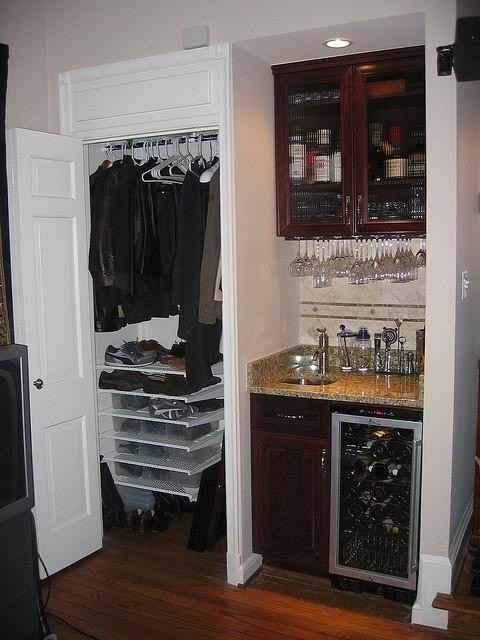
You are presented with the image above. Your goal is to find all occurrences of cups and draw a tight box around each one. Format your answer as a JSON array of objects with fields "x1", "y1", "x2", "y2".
[
  {"x1": 356, "y1": 327, "x2": 372, "y2": 371},
  {"x1": 337, "y1": 330, "x2": 355, "y2": 372}
]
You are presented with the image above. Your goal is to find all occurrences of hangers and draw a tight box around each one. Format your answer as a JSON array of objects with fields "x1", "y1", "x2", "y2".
[{"x1": 90, "y1": 133, "x2": 222, "y2": 197}]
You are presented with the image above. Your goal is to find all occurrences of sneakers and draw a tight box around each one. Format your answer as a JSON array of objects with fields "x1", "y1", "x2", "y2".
[
  {"x1": 149, "y1": 397, "x2": 223, "y2": 421},
  {"x1": 120, "y1": 418, "x2": 212, "y2": 440},
  {"x1": 118, "y1": 442, "x2": 169, "y2": 457},
  {"x1": 104, "y1": 342, "x2": 157, "y2": 367},
  {"x1": 132, "y1": 339, "x2": 171, "y2": 361},
  {"x1": 143, "y1": 373, "x2": 221, "y2": 396},
  {"x1": 160, "y1": 341, "x2": 186, "y2": 371},
  {"x1": 118, "y1": 462, "x2": 171, "y2": 481},
  {"x1": 99, "y1": 369, "x2": 147, "y2": 392},
  {"x1": 125, "y1": 510, "x2": 172, "y2": 536},
  {"x1": 120, "y1": 395, "x2": 150, "y2": 410}
]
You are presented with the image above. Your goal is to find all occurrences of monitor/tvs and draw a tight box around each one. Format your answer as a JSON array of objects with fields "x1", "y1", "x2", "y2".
[{"x1": 0, "y1": 343, "x2": 35, "y2": 523}]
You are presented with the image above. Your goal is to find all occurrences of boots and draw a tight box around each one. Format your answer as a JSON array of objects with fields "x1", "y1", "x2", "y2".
[{"x1": 100, "y1": 455, "x2": 125, "y2": 532}]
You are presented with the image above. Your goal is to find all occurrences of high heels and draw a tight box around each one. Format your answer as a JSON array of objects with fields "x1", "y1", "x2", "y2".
[{"x1": 153, "y1": 491, "x2": 185, "y2": 521}]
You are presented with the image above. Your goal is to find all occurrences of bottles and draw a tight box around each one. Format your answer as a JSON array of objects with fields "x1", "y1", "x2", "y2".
[
  {"x1": 343, "y1": 428, "x2": 413, "y2": 539},
  {"x1": 290, "y1": 117, "x2": 426, "y2": 184}
]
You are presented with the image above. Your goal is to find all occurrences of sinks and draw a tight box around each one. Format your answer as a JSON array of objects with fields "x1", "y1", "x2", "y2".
[{"x1": 278, "y1": 371, "x2": 336, "y2": 387}]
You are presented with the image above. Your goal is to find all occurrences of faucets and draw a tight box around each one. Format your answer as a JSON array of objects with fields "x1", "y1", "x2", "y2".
[{"x1": 313, "y1": 328, "x2": 330, "y2": 376}]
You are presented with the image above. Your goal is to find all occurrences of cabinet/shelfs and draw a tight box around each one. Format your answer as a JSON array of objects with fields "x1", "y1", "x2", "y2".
[
  {"x1": 253, "y1": 396, "x2": 421, "y2": 598},
  {"x1": 94, "y1": 364, "x2": 224, "y2": 500},
  {"x1": 266, "y1": 45, "x2": 425, "y2": 240}
]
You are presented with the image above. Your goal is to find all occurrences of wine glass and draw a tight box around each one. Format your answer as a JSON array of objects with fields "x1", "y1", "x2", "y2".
[{"x1": 289, "y1": 235, "x2": 426, "y2": 290}]
